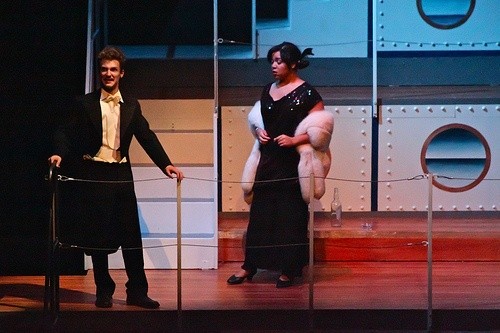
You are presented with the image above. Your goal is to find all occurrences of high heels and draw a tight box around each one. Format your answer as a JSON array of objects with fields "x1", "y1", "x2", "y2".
[
  {"x1": 227, "y1": 266, "x2": 257, "y2": 284},
  {"x1": 276, "y1": 276, "x2": 292, "y2": 287}
]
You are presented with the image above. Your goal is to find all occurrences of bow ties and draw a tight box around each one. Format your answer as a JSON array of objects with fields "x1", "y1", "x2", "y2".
[{"x1": 107, "y1": 94, "x2": 121, "y2": 107}]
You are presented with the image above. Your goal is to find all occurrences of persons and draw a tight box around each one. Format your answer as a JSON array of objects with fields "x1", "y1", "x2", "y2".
[
  {"x1": 227, "y1": 41, "x2": 333, "y2": 287},
  {"x1": 47, "y1": 47, "x2": 183, "y2": 307}
]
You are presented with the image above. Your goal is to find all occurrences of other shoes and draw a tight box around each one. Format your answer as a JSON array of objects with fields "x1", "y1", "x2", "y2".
[
  {"x1": 125, "y1": 293, "x2": 160, "y2": 308},
  {"x1": 95, "y1": 295, "x2": 113, "y2": 308}
]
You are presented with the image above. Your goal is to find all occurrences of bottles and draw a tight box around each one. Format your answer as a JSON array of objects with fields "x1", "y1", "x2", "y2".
[{"x1": 331, "y1": 187, "x2": 342, "y2": 227}]
